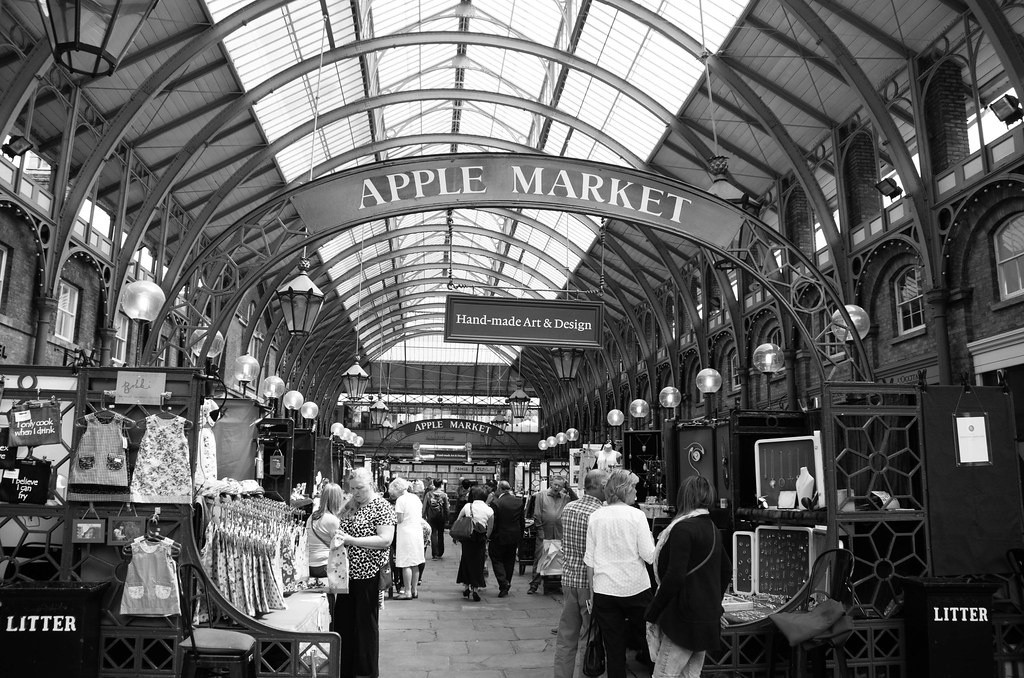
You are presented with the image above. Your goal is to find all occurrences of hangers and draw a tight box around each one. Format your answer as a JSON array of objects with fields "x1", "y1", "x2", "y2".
[
  {"x1": 75, "y1": 390, "x2": 194, "y2": 430},
  {"x1": 123, "y1": 514, "x2": 182, "y2": 557},
  {"x1": 206, "y1": 494, "x2": 305, "y2": 537}
]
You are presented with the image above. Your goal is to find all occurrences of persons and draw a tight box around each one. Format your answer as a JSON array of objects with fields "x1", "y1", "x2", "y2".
[
  {"x1": 452, "y1": 487, "x2": 494, "y2": 602},
  {"x1": 489, "y1": 480, "x2": 524, "y2": 598},
  {"x1": 583, "y1": 470, "x2": 655, "y2": 678},
  {"x1": 305, "y1": 483, "x2": 343, "y2": 578},
  {"x1": 554, "y1": 469, "x2": 609, "y2": 678},
  {"x1": 594, "y1": 444, "x2": 622, "y2": 470},
  {"x1": 422, "y1": 478, "x2": 450, "y2": 560},
  {"x1": 643, "y1": 475, "x2": 733, "y2": 677},
  {"x1": 337, "y1": 467, "x2": 398, "y2": 677},
  {"x1": 527, "y1": 474, "x2": 579, "y2": 594},
  {"x1": 388, "y1": 477, "x2": 431, "y2": 600},
  {"x1": 453, "y1": 479, "x2": 525, "y2": 540}
]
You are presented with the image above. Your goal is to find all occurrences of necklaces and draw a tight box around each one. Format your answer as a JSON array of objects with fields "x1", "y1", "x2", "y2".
[{"x1": 764, "y1": 448, "x2": 807, "y2": 488}]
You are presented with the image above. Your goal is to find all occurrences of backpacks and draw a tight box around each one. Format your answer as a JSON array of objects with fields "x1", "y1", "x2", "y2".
[{"x1": 427, "y1": 489, "x2": 445, "y2": 519}]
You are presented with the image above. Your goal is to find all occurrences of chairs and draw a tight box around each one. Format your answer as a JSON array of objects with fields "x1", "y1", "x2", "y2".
[
  {"x1": 765, "y1": 548, "x2": 855, "y2": 678},
  {"x1": 176, "y1": 562, "x2": 259, "y2": 678}
]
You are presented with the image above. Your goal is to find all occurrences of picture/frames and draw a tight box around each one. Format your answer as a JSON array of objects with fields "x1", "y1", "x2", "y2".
[
  {"x1": 72, "y1": 519, "x2": 105, "y2": 544},
  {"x1": 108, "y1": 515, "x2": 146, "y2": 545}
]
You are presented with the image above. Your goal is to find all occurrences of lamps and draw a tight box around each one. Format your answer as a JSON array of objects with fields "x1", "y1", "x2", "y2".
[
  {"x1": 483, "y1": 344, "x2": 532, "y2": 446},
  {"x1": 989, "y1": 93, "x2": 1024, "y2": 126},
  {"x1": 875, "y1": 178, "x2": 902, "y2": 203},
  {"x1": 273, "y1": 11, "x2": 326, "y2": 337},
  {"x1": 607, "y1": 305, "x2": 870, "y2": 440},
  {"x1": 709, "y1": 296, "x2": 720, "y2": 308},
  {"x1": 35, "y1": 0, "x2": 160, "y2": 76},
  {"x1": 330, "y1": 223, "x2": 397, "y2": 447},
  {"x1": 699, "y1": 0, "x2": 765, "y2": 271},
  {"x1": 539, "y1": 428, "x2": 579, "y2": 462},
  {"x1": 2, "y1": 135, "x2": 34, "y2": 158},
  {"x1": 120, "y1": 278, "x2": 317, "y2": 427},
  {"x1": 545, "y1": 214, "x2": 585, "y2": 379}
]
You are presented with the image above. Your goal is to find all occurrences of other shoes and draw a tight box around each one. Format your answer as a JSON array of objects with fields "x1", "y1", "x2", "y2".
[
  {"x1": 393, "y1": 593, "x2": 412, "y2": 599},
  {"x1": 528, "y1": 586, "x2": 535, "y2": 594},
  {"x1": 413, "y1": 591, "x2": 418, "y2": 598},
  {"x1": 499, "y1": 582, "x2": 511, "y2": 598}
]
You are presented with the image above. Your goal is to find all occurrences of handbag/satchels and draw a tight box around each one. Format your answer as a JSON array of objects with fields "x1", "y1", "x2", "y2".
[
  {"x1": 645, "y1": 620, "x2": 662, "y2": 663},
  {"x1": 448, "y1": 503, "x2": 472, "y2": 539},
  {"x1": 583, "y1": 606, "x2": 605, "y2": 677}
]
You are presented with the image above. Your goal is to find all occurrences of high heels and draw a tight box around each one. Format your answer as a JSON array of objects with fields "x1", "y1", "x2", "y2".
[
  {"x1": 462, "y1": 588, "x2": 470, "y2": 599},
  {"x1": 473, "y1": 592, "x2": 481, "y2": 602}
]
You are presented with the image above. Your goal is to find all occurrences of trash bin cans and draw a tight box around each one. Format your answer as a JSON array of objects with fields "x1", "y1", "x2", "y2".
[
  {"x1": 895, "y1": 575, "x2": 1004, "y2": 678},
  {"x1": 0, "y1": 581, "x2": 112, "y2": 678}
]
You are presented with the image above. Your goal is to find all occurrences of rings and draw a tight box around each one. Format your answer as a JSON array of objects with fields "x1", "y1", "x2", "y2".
[{"x1": 738, "y1": 534, "x2": 808, "y2": 592}]
